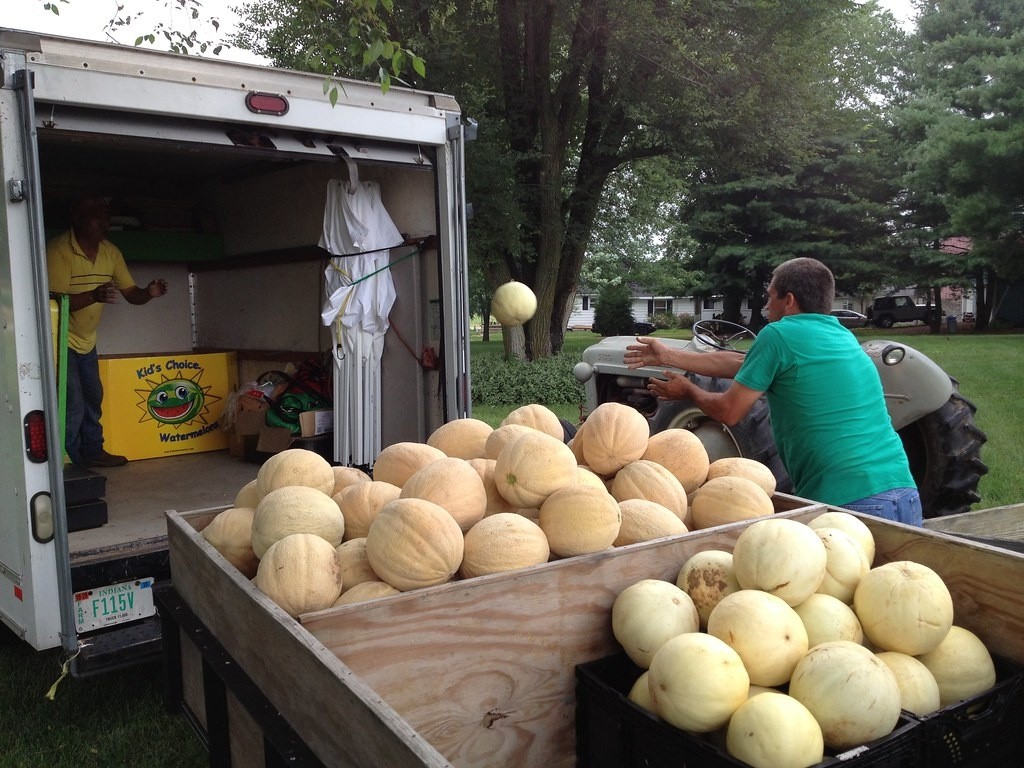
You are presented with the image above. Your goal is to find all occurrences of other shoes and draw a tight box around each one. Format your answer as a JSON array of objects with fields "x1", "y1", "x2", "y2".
[{"x1": 75, "y1": 449, "x2": 128, "y2": 467}]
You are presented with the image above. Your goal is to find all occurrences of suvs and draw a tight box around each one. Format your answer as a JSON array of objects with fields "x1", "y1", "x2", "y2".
[{"x1": 868, "y1": 295, "x2": 946, "y2": 329}]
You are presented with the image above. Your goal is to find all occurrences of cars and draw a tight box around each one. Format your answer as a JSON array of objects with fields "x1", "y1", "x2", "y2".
[{"x1": 829, "y1": 308, "x2": 870, "y2": 329}]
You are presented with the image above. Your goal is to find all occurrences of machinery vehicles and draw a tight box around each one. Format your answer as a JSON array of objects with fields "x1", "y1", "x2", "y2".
[{"x1": 547, "y1": 318, "x2": 988, "y2": 519}]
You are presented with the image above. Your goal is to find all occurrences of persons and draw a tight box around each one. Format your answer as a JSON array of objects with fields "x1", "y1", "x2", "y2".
[
  {"x1": 623, "y1": 257, "x2": 923, "y2": 528},
  {"x1": 46, "y1": 193, "x2": 168, "y2": 468}
]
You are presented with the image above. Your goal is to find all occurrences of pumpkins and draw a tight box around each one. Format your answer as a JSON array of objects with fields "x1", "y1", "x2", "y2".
[
  {"x1": 198, "y1": 401, "x2": 776, "y2": 618},
  {"x1": 491, "y1": 279, "x2": 537, "y2": 327}
]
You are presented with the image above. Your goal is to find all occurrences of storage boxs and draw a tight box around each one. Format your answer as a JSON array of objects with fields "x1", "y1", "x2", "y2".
[{"x1": 573, "y1": 649, "x2": 1024, "y2": 768}]
